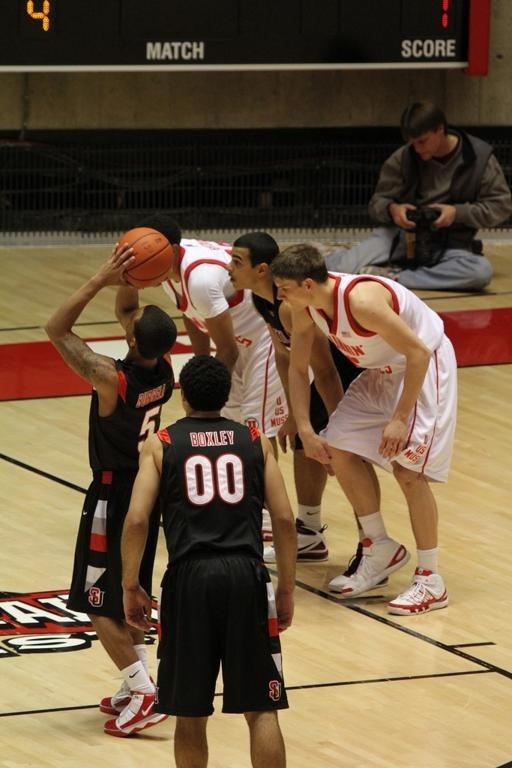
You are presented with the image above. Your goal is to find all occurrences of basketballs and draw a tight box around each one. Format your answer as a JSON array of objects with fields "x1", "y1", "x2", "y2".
[{"x1": 117, "y1": 227, "x2": 174, "y2": 288}]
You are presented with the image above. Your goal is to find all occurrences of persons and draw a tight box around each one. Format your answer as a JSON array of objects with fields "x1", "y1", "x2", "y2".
[
  {"x1": 135, "y1": 213, "x2": 315, "y2": 461},
  {"x1": 43, "y1": 240, "x2": 175, "y2": 738},
  {"x1": 326, "y1": 98, "x2": 511, "y2": 291},
  {"x1": 119, "y1": 351, "x2": 301, "y2": 768},
  {"x1": 269, "y1": 241, "x2": 460, "y2": 617},
  {"x1": 226, "y1": 230, "x2": 389, "y2": 594}
]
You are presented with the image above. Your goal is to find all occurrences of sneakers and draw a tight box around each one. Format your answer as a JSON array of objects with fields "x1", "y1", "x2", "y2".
[
  {"x1": 262, "y1": 519, "x2": 330, "y2": 563},
  {"x1": 386, "y1": 569, "x2": 449, "y2": 615},
  {"x1": 100, "y1": 682, "x2": 133, "y2": 715},
  {"x1": 327, "y1": 536, "x2": 411, "y2": 598},
  {"x1": 104, "y1": 693, "x2": 167, "y2": 736}
]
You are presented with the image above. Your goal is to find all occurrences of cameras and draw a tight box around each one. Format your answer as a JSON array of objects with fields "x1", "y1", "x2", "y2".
[{"x1": 406, "y1": 208, "x2": 441, "y2": 269}]
